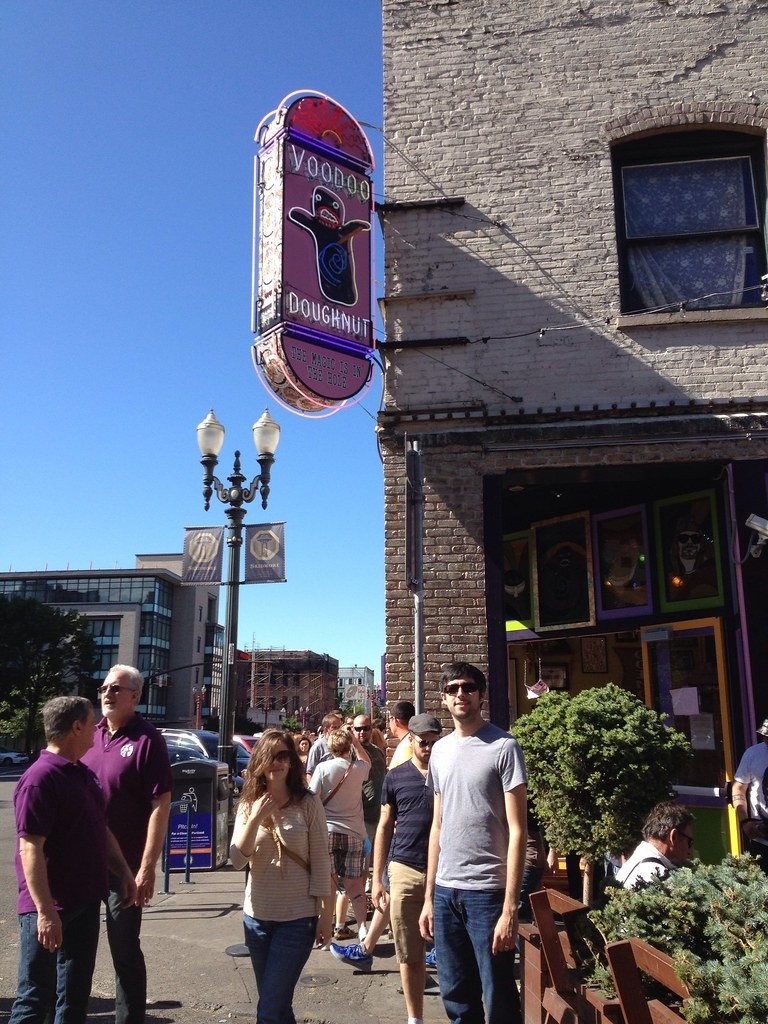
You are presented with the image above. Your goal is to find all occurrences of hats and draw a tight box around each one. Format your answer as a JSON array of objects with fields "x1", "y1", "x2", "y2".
[
  {"x1": 408, "y1": 713, "x2": 443, "y2": 735},
  {"x1": 755, "y1": 719, "x2": 768, "y2": 737}
]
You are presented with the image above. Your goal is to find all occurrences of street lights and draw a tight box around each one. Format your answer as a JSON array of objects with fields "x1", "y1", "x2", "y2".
[
  {"x1": 300, "y1": 705, "x2": 309, "y2": 729},
  {"x1": 195, "y1": 407, "x2": 281, "y2": 835}
]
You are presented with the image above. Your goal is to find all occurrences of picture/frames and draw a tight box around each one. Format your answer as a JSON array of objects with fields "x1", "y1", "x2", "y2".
[
  {"x1": 536, "y1": 662, "x2": 570, "y2": 690},
  {"x1": 580, "y1": 636, "x2": 609, "y2": 673},
  {"x1": 655, "y1": 489, "x2": 726, "y2": 613},
  {"x1": 531, "y1": 510, "x2": 598, "y2": 632},
  {"x1": 671, "y1": 649, "x2": 695, "y2": 671},
  {"x1": 501, "y1": 527, "x2": 536, "y2": 633},
  {"x1": 592, "y1": 501, "x2": 655, "y2": 620}
]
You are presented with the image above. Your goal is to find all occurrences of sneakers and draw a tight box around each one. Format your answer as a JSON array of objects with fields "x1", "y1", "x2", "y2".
[
  {"x1": 425, "y1": 947, "x2": 437, "y2": 968},
  {"x1": 330, "y1": 943, "x2": 373, "y2": 973}
]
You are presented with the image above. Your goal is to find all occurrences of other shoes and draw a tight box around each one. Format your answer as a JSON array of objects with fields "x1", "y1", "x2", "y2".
[
  {"x1": 396, "y1": 972, "x2": 441, "y2": 995},
  {"x1": 358, "y1": 927, "x2": 369, "y2": 942},
  {"x1": 346, "y1": 902, "x2": 355, "y2": 918},
  {"x1": 312, "y1": 937, "x2": 332, "y2": 951},
  {"x1": 334, "y1": 924, "x2": 357, "y2": 941},
  {"x1": 365, "y1": 892, "x2": 374, "y2": 913}
]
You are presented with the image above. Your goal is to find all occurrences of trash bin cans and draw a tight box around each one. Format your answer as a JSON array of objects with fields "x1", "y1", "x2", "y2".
[{"x1": 161, "y1": 760, "x2": 234, "y2": 872}]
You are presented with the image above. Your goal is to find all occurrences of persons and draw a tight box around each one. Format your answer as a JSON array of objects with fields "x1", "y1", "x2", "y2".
[
  {"x1": 80, "y1": 664, "x2": 174, "y2": 1024},
  {"x1": 296, "y1": 710, "x2": 386, "y2": 950},
  {"x1": 371, "y1": 713, "x2": 443, "y2": 1024},
  {"x1": 514, "y1": 800, "x2": 559, "y2": 951},
  {"x1": 8, "y1": 696, "x2": 138, "y2": 1024},
  {"x1": 330, "y1": 701, "x2": 416, "y2": 972},
  {"x1": 229, "y1": 729, "x2": 332, "y2": 1024},
  {"x1": 419, "y1": 662, "x2": 528, "y2": 1024},
  {"x1": 732, "y1": 718, "x2": 768, "y2": 877},
  {"x1": 614, "y1": 801, "x2": 697, "y2": 942}
]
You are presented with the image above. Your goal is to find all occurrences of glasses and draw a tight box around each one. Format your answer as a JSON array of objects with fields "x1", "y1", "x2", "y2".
[
  {"x1": 340, "y1": 718, "x2": 344, "y2": 722},
  {"x1": 411, "y1": 735, "x2": 436, "y2": 749},
  {"x1": 443, "y1": 682, "x2": 480, "y2": 694},
  {"x1": 387, "y1": 715, "x2": 401, "y2": 723},
  {"x1": 353, "y1": 725, "x2": 371, "y2": 732},
  {"x1": 666, "y1": 828, "x2": 694, "y2": 849},
  {"x1": 97, "y1": 685, "x2": 135, "y2": 695},
  {"x1": 261, "y1": 749, "x2": 291, "y2": 764}
]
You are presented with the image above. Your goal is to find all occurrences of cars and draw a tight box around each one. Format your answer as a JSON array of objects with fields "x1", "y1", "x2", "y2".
[
  {"x1": 154, "y1": 727, "x2": 263, "y2": 797},
  {"x1": 0, "y1": 746, "x2": 30, "y2": 767}
]
